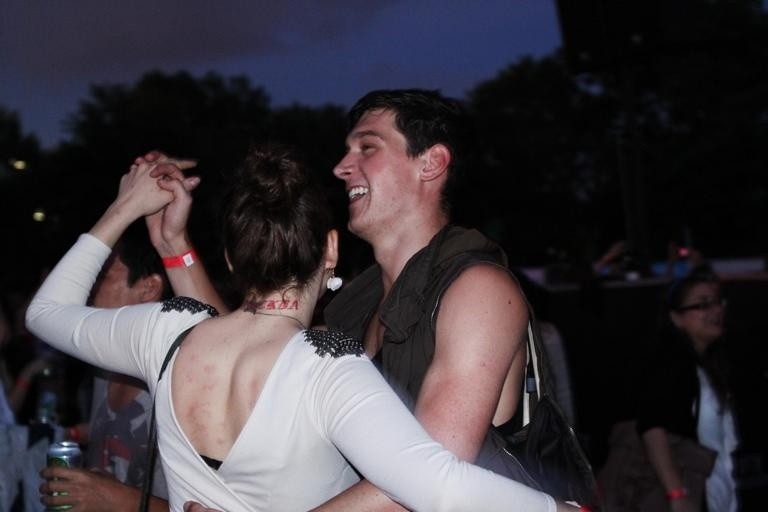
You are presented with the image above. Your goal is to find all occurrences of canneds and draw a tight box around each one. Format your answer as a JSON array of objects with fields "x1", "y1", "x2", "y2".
[
  {"x1": 35, "y1": 390, "x2": 57, "y2": 425},
  {"x1": 47, "y1": 441, "x2": 83, "y2": 511}
]
